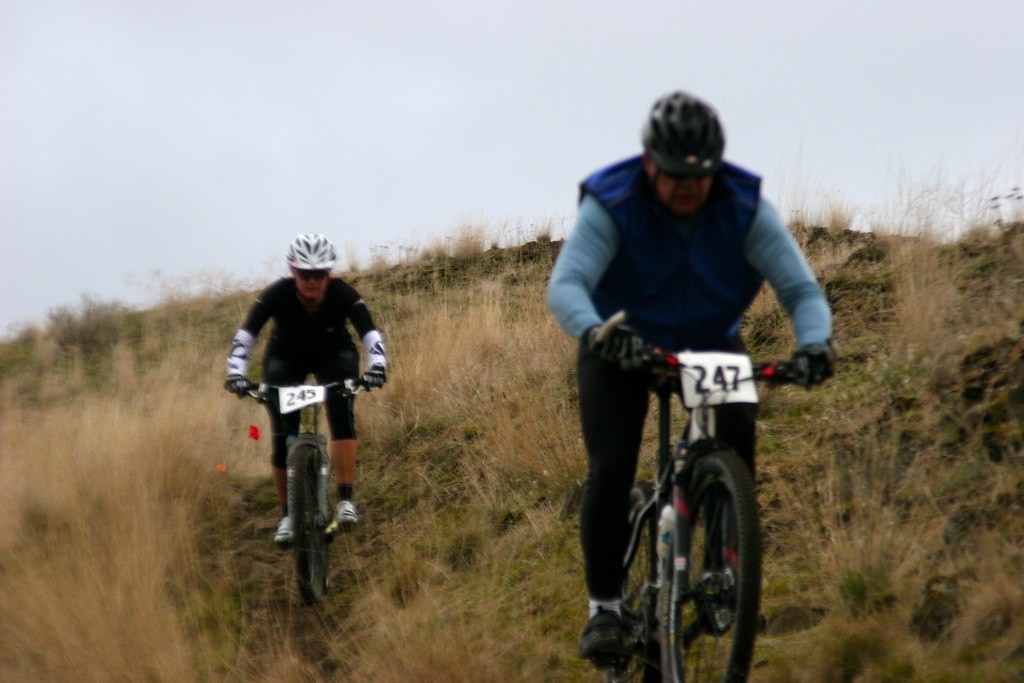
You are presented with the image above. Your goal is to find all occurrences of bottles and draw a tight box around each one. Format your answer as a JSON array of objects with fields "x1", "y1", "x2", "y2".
[{"x1": 655, "y1": 505, "x2": 676, "y2": 559}]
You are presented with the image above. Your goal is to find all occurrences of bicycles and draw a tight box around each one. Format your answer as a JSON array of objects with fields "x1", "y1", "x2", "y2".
[
  {"x1": 590, "y1": 318, "x2": 834, "y2": 683},
  {"x1": 240, "y1": 378, "x2": 373, "y2": 605}
]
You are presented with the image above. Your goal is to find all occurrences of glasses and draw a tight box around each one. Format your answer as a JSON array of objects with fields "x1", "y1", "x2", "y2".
[{"x1": 293, "y1": 268, "x2": 327, "y2": 281}]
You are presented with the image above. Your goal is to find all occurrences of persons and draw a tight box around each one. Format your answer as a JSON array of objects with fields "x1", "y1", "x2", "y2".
[
  {"x1": 224, "y1": 232, "x2": 388, "y2": 542},
  {"x1": 546, "y1": 91, "x2": 840, "y2": 672}
]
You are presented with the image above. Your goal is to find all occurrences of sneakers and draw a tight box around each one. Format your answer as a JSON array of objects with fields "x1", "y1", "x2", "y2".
[
  {"x1": 333, "y1": 500, "x2": 359, "y2": 526},
  {"x1": 579, "y1": 608, "x2": 625, "y2": 658},
  {"x1": 694, "y1": 571, "x2": 738, "y2": 634},
  {"x1": 274, "y1": 516, "x2": 295, "y2": 545}
]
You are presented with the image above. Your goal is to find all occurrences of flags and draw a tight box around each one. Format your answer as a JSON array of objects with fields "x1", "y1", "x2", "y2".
[{"x1": 249, "y1": 424, "x2": 260, "y2": 440}]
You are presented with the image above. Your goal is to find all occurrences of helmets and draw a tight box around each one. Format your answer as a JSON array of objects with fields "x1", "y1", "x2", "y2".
[
  {"x1": 285, "y1": 233, "x2": 336, "y2": 270},
  {"x1": 644, "y1": 92, "x2": 725, "y2": 168}
]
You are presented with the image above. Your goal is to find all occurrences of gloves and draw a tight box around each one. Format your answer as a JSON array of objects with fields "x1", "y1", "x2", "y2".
[
  {"x1": 224, "y1": 375, "x2": 251, "y2": 399},
  {"x1": 788, "y1": 343, "x2": 835, "y2": 390},
  {"x1": 361, "y1": 370, "x2": 383, "y2": 392},
  {"x1": 587, "y1": 321, "x2": 645, "y2": 372}
]
